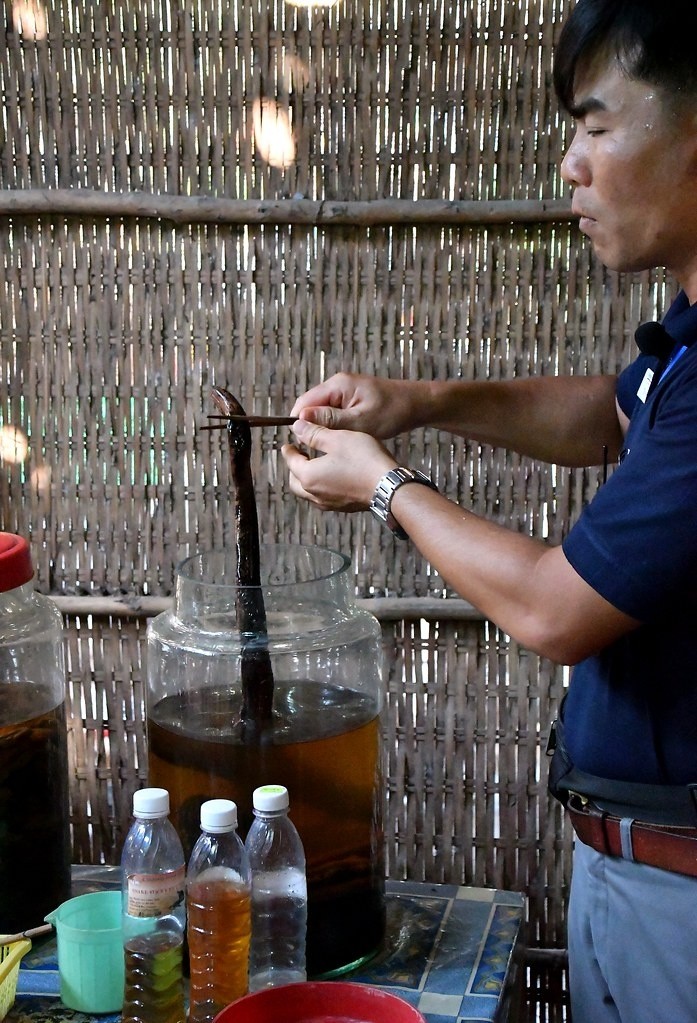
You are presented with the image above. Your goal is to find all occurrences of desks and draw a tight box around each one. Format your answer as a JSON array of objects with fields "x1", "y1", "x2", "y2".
[{"x1": 0, "y1": 860, "x2": 530, "y2": 1023}]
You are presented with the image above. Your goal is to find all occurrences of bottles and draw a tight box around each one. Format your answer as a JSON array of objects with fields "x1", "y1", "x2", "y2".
[
  {"x1": 1, "y1": 530, "x2": 74, "y2": 954},
  {"x1": 245, "y1": 784, "x2": 307, "y2": 994},
  {"x1": 120, "y1": 788, "x2": 187, "y2": 1023},
  {"x1": 145, "y1": 541, "x2": 389, "y2": 981},
  {"x1": 186, "y1": 800, "x2": 251, "y2": 1023}
]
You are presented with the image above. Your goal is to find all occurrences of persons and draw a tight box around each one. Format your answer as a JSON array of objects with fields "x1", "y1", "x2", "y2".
[{"x1": 283, "y1": 0, "x2": 696, "y2": 1023}]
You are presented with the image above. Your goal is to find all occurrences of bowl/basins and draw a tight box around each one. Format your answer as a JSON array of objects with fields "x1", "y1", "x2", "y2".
[{"x1": 215, "y1": 981, "x2": 426, "y2": 1023}]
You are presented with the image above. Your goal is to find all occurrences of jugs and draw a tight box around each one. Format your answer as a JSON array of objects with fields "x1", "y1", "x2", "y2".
[{"x1": 45, "y1": 891, "x2": 126, "y2": 1013}]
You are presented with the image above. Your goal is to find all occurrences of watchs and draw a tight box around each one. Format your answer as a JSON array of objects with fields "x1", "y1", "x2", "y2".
[{"x1": 370, "y1": 468, "x2": 438, "y2": 542}]
